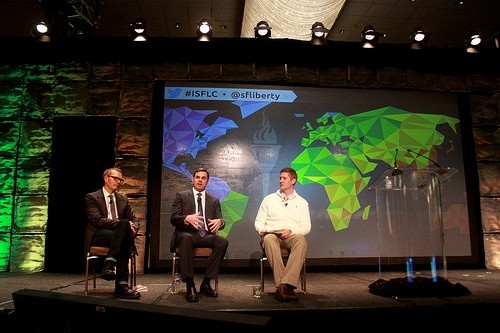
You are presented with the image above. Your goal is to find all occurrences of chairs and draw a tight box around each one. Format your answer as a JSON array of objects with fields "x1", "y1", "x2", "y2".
[
  {"x1": 261, "y1": 248, "x2": 306, "y2": 295},
  {"x1": 171, "y1": 248, "x2": 218, "y2": 296},
  {"x1": 84, "y1": 246, "x2": 137, "y2": 296}
]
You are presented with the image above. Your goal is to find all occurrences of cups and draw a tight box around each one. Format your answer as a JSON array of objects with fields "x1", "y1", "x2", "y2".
[{"x1": 252, "y1": 286, "x2": 261, "y2": 298}]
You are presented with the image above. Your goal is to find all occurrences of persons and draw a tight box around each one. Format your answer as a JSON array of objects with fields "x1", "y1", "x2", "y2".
[
  {"x1": 170, "y1": 168, "x2": 228, "y2": 303},
  {"x1": 254, "y1": 167, "x2": 311, "y2": 302},
  {"x1": 86, "y1": 168, "x2": 142, "y2": 299}
]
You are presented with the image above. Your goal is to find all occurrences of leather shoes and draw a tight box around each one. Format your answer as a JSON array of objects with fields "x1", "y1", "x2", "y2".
[
  {"x1": 274, "y1": 288, "x2": 290, "y2": 301},
  {"x1": 100, "y1": 265, "x2": 116, "y2": 280},
  {"x1": 288, "y1": 290, "x2": 299, "y2": 301},
  {"x1": 114, "y1": 286, "x2": 141, "y2": 298},
  {"x1": 185, "y1": 287, "x2": 200, "y2": 301},
  {"x1": 200, "y1": 284, "x2": 218, "y2": 297}
]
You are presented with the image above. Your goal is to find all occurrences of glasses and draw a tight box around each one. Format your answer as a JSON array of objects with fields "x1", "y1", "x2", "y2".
[{"x1": 107, "y1": 174, "x2": 124, "y2": 184}]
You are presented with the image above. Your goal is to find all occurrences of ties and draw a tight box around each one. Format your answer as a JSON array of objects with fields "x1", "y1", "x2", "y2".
[
  {"x1": 108, "y1": 195, "x2": 117, "y2": 220},
  {"x1": 197, "y1": 193, "x2": 206, "y2": 238}
]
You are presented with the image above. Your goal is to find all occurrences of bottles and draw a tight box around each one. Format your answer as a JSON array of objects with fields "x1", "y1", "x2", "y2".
[{"x1": 174, "y1": 273, "x2": 179, "y2": 294}]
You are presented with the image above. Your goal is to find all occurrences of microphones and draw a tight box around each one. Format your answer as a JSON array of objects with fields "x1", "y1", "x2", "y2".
[
  {"x1": 110, "y1": 200, "x2": 112, "y2": 203},
  {"x1": 392, "y1": 149, "x2": 403, "y2": 176},
  {"x1": 286, "y1": 203, "x2": 288, "y2": 206},
  {"x1": 407, "y1": 149, "x2": 447, "y2": 175}
]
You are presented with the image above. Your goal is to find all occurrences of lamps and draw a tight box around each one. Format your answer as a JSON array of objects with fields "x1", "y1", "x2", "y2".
[
  {"x1": 311, "y1": 22, "x2": 330, "y2": 40},
  {"x1": 409, "y1": 27, "x2": 425, "y2": 43},
  {"x1": 254, "y1": 20, "x2": 271, "y2": 39},
  {"x1": 360, "y1": 25, "x2": 381, "y2": 43},
  {"x1": 466, "y1": 31, "x2": 482, "y2": 48},
  {"x1": 130, "y1": 18, "x2": 148, "y2": 37},
  {"x1": 36, "y1": 17, "x2": 51, "y2": 34},
  {"x1": 196, "y1": 19, "x2": 213, "y2": 38}
]
